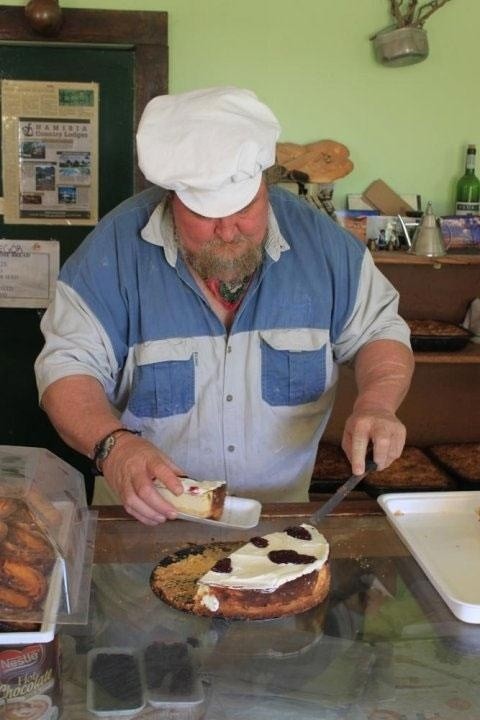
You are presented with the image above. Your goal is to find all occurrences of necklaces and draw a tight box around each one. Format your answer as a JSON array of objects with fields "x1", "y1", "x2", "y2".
[{"x1": 200, "y1": 274, "x2": 254, "y2": 310}]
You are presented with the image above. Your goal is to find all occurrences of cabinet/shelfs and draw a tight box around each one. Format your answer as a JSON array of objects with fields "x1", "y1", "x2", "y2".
[{"x1": 370, "y1": 250, "x2": 480, "y2": 364}]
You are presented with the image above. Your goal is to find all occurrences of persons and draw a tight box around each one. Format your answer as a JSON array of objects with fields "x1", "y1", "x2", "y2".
[{"x1": 28, "y1": 79, "x2": 422, "y2": 660}]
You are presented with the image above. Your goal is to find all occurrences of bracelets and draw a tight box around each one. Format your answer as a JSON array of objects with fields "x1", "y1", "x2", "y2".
[{"x1": 92, "y1": 426, "x2": 144, "y2": 478}]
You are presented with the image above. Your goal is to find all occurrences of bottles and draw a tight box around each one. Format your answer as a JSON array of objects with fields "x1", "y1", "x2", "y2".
[{"x1": 454, "y1": 143, "x2": 479, "y2": 217}]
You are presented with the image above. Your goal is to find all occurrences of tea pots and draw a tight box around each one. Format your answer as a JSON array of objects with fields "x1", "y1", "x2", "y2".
[{"x1": 396, "y1": 201, "x2": 453, "y2": 257}]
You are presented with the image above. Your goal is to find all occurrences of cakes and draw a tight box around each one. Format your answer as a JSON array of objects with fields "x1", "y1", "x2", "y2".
[{"x1": 193, "y1": 521, "x2": 330, "y2": 619}]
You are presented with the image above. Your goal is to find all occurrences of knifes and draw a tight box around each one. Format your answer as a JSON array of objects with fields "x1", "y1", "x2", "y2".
[{"x1": 307, "y1": 462, "x2": 377, "y2": 530}]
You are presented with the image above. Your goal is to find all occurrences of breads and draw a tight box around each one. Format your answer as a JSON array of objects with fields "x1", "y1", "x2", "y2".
[
  {"x1": 276, "y1": 140, "x2": 354, "y2": 183},
  {"x1": 151, "y1": 473, "x2": 227, "y2": 519},
  {"x1": 0, "y1": 475, "x2": 67, "y2": 631}
]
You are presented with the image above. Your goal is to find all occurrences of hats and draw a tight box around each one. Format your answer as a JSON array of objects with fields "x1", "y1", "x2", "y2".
[{"x1": 135, "y1": 85, "x2": 279, "y2": 219}]
